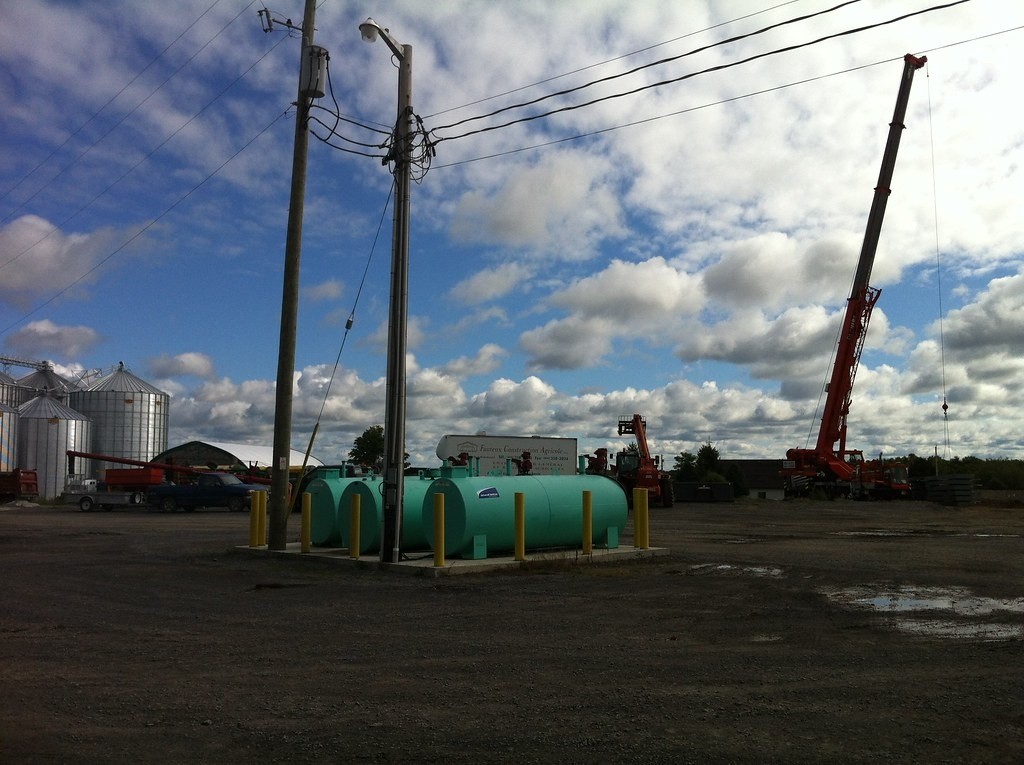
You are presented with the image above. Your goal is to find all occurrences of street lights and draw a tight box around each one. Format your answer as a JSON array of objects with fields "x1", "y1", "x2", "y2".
[{"x1": 359, "y1": 15, "x2": 411, "y2": 567}]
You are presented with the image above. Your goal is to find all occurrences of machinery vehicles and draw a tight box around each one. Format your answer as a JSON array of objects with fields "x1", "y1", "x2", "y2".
[
  {"x1": 586, "y1": 414, "x2": 677, "y2": 509},
  {"x1": 778, "y1": 53, "x2": 950, "y2": 504}
]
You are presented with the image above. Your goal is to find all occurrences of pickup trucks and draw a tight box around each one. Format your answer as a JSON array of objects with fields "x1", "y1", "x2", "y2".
[{"x1": 170, "y1": 473, "x2": 269, "y2": 513}]
